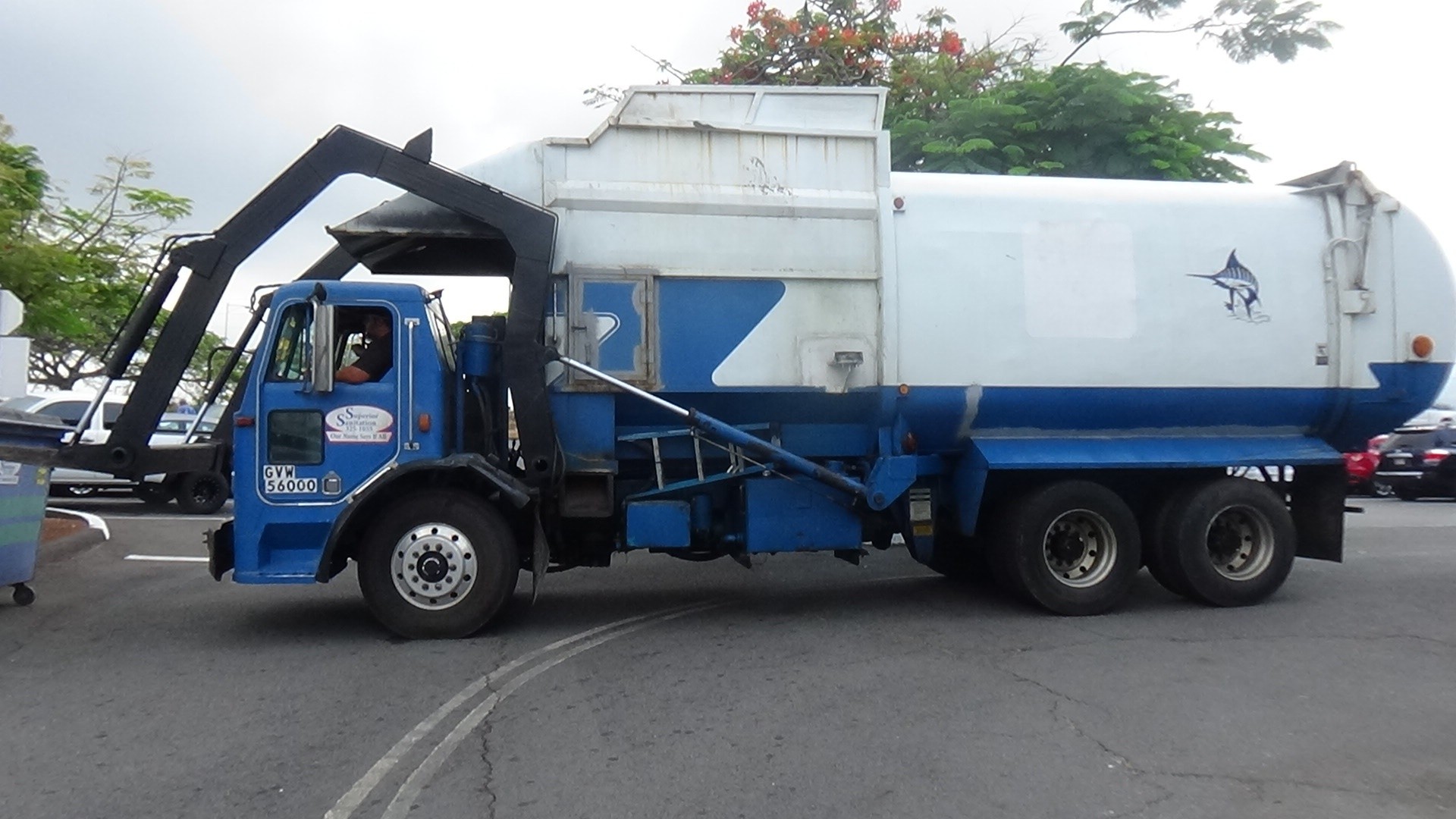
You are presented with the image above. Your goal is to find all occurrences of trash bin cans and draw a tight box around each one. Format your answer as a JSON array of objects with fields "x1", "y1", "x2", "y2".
[{"x1": 0, "y1": 405, "x2": 76, "y2": 605}]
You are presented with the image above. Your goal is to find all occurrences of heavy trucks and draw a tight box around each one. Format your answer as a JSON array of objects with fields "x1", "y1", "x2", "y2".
[{"x1": 0, "y1": 85, "x2": 1456, "y2": 641}]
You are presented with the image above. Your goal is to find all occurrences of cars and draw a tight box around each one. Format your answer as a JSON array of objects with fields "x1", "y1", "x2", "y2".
[
  {"x1": 1343, "y1": 417, "x2": 1455, "y2": 501},
  {"x1": 0, "y1": 396, "x2": 220, "y2": 498}
]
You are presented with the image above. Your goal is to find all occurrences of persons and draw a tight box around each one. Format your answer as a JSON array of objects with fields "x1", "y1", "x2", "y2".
[
  {"x1": 335, "y1": 306, "x2": 394, "y2": 385},
  {"x1": 177, "y1": 399, "x2": 195, "y2": 415}
]
